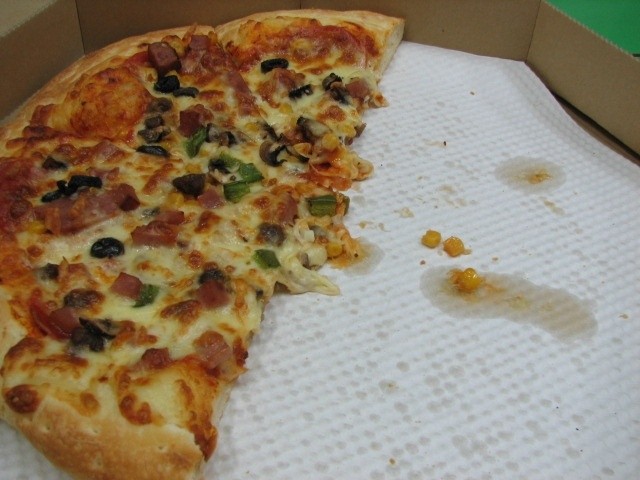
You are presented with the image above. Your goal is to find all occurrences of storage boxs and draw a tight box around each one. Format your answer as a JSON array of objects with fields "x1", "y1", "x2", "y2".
[{"x1": 0, "y1": 0, "x2": 640, "y2": 479}]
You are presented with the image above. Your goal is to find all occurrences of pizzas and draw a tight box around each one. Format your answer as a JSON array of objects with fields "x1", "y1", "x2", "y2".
[{"x1": 0, "y1": 9, "x2": 405, "y2": 476}]
